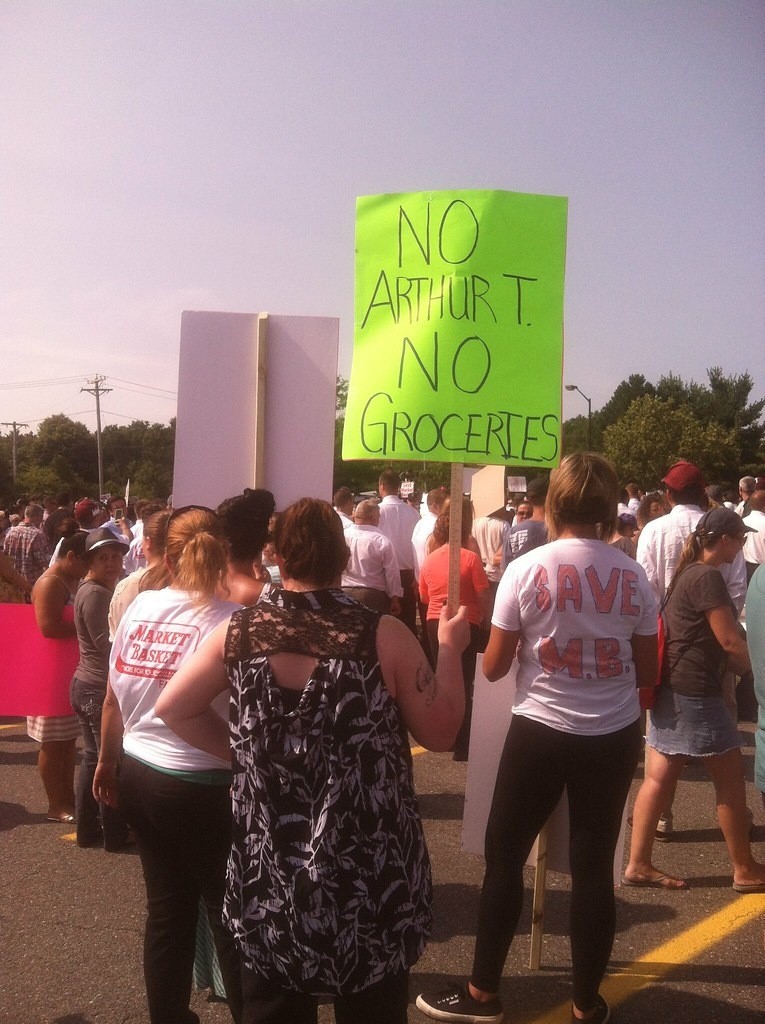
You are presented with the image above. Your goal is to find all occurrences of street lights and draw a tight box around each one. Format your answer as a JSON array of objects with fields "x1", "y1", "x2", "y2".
[{"x1": 565, "y1": 385, "x2": 591, "y2": 452}]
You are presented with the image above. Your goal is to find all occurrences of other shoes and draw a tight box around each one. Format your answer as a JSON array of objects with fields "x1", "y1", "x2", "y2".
[
  {"x1": 453, "y1": 750, "x2": 469, "y2": 761},
  {"x1": 626, "y1": 816, "x2": 673, "y2": 842},
  {"x1": 76, "y1": 839, "x2": 104, "y2": 847},
  {"x1": 124, "y1": 830, "x2": 137, "y2": 844}
]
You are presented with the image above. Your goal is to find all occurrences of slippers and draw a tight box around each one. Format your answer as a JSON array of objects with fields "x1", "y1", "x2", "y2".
[
  {"x1": 732, "y1": 882, "x2": 765, "y2": 893},
  {"x1": 621, "y1": 870, "x2": 687, "y2": 889},
  {"x1": 46, "y1": 812, "x2": 78, "y2": 824}
]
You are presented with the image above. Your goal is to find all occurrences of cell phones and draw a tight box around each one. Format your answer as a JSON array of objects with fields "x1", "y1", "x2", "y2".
[{"x1": 114, "y1": 508, "x2": 124, "y2": 526}]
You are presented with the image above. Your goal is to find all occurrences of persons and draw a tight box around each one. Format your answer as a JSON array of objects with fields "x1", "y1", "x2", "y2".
[
  {"x1": 334, "y1": 473, "x2": 550, "y2": 672},
  {"x1": 616, "y1": 504, "x2": 765, "y2": 892},
  {"x1": 414, "y1": 455, "x2": 657, "y2": 1024},
  {"x1": 416, "y1": 508, "x2": 491, "y2": 686},
  {"x1": 68, "y1": 528, "x2": 138, "y2": 851},
  {"x1": 150, "y1": 497, "x2": 471, "y2": 1024},
  {"x1": 93, "y1": 508, "x2": 245, "y2": 1024},
  {"x1": 0, "y1": 495, "x2": 405, "y2": 824},
  {"x1": 613, "y1": 475, "x2": 765, "y2": 839},
  {"x1": 497, "y1": 477, "x2": 553, "y2": 578}
]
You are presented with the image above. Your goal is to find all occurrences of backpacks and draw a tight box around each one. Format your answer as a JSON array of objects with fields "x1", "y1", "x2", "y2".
[{"x1": 639, "y1": 562, "x2": 709, "y2": 709}]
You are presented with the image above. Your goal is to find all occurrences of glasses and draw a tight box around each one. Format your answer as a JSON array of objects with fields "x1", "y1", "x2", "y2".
[
  {"x1": 729, "y1": 535, "x2": 748, "y2": 546},
  {"x1": 165, "y1": 504, "x2": 217, "y2": 534},
  {"x1": 516, "y1": 512, "x2": 533, "y2": 517}
]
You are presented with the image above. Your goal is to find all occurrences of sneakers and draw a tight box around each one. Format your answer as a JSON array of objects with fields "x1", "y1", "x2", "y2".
[
  {"x1": 415, "y1": 982, "x2": 505, "y2": 1024},
  {"x1": 571, "y1": 993, "x2": 611, "y2": 1024}
]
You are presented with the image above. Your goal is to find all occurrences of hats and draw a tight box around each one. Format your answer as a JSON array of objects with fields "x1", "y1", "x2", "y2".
[
  {"x1": 619, "y1": 513, "x2": 640, "y2": 531},
  {"x1": 79, "y1": 526, "x2": 130, "y2": 561},
  {"x1": 659, "y1": 460, "x2": 701, "y2": 492},
  {"x1": 755, "y1": 477, "x2": 765, "y2": 483},
  {"x1": 408, "y1": 493, "x2": 416, "y2": 500},
  {"x1": 527, "y1": 478, "x2": 547, "y2": 493},
  {"x1": 695, "y1": 507, "x2": 758, "y2": 538}
]
